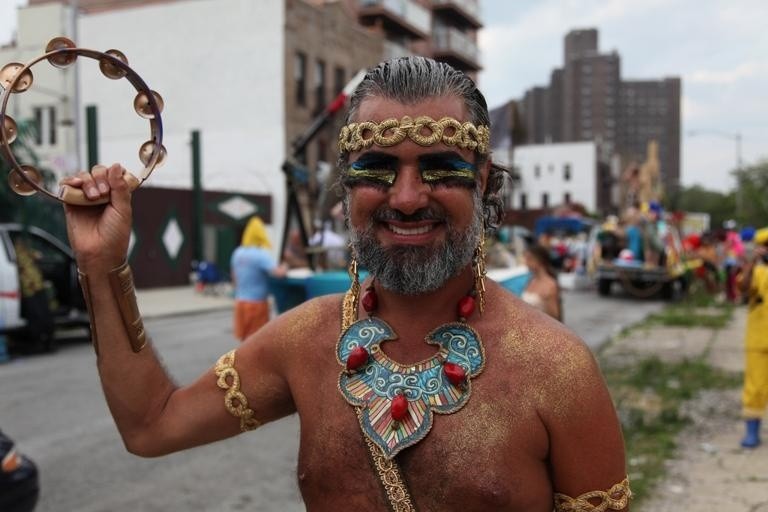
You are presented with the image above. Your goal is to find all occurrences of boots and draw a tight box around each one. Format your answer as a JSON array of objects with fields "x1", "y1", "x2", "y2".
[{"x1": 741, "y1": 421, "x2": 759, "y2": 446}]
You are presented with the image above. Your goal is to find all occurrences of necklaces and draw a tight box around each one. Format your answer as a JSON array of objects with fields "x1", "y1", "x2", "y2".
[{"x1": 334, "y1": 270, "x2": 486, "y2": 462}]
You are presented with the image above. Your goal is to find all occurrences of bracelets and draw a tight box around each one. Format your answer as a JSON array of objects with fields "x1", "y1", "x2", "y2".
[{"x1": 76, "y1": 258, "x2": 148, "y2": 358}]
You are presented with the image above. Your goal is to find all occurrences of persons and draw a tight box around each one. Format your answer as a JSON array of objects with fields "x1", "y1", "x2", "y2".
[
  {"x1": 520, "y1": 243, "x2": 562, "y2": 325},
  {"x1": 59, "y1": 55, "x2": 633, "y2": 512},
  {"x1": 228, "y1": 216, "x2": 289, "y2": 344},
  {"x1": 538, "y1": 200, "x2": 755, "y2": 305},
  {"x1": 322, "y1": 200, "x2": 352, "y2": 271},
  {"x1": 740, "y1": 227, "x2": 768, "y2": 447}
]
